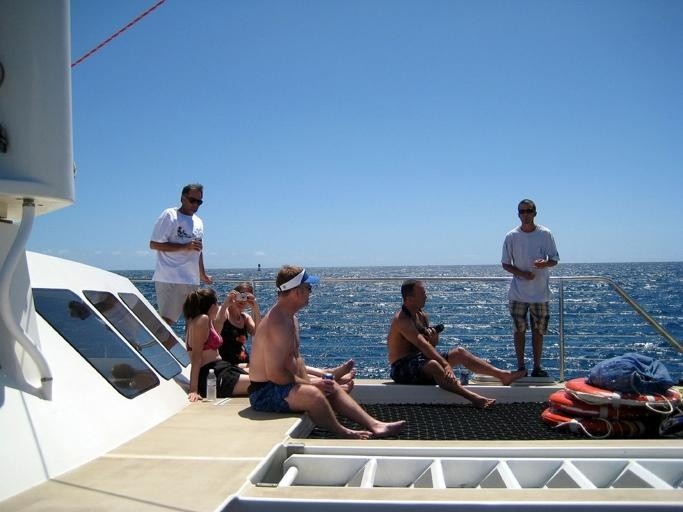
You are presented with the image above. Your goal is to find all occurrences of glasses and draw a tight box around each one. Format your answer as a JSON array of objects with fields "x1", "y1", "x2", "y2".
[{"x1": 182, "y1": 193, "x2": 203, "y2": 206}]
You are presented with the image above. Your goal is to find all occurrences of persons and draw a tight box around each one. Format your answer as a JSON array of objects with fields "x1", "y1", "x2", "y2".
[
  {"x1": 183, "y1": 288, "x2": 353, "y2": 402},
  {"x1": 150, "y1": 182, "x2": 213, "y2": 325},
  {"x1": 111, "y1": 365, "x2": 160, "y2": 391},
  {"x1": 248, "y1": 264, "x2": 407, "y2": 440},
  {"x1": 501, "y1": 199, "x2": 559, "y2": 377},
  {"x1": 387, "y1": 279, "x2": 528, "y2": 410},
  {"x1": 212, "y1": 282, "x2": 356, "y2": 384}
]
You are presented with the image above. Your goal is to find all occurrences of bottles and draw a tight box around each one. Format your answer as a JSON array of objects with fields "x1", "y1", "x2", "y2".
[
  {"x1": 460, "y1": 368, "x2": 469, "y2": 386},
  {"x1": 205, "y1": 368, "x2": 217, "y2": 400}
]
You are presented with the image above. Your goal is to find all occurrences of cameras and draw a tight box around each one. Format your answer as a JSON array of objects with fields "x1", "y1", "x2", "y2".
[
  {"x1": 231, "y1": 290, "x2": 249, "y2": 303},
  {"x1": 419, "y1": 324, "x2": 446, "y2": 336}
]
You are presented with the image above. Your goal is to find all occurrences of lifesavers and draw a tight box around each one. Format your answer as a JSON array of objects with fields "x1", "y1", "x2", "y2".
[
  {"x1": 565, "y1": 378, "x2": 682, "y2": 415},
  {"x1": 541, "y1": 408, "x2": 649, "y2": 438},
  {"x1": 547, "y1": 390, "x2": 649, "y2": 420}
]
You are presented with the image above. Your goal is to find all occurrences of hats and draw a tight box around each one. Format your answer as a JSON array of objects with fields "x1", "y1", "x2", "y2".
[{"x1": 274, "y1": 268, "x2": 321, "y2": 292}]
